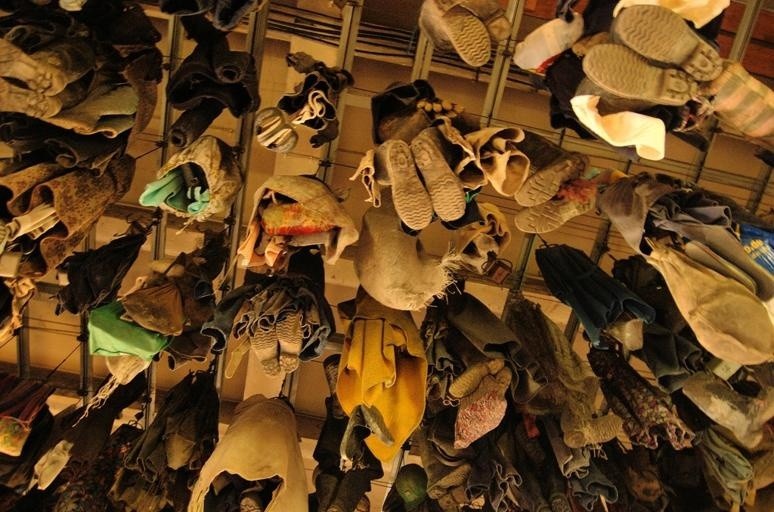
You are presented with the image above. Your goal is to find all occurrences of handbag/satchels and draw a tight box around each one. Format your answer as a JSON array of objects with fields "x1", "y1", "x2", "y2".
[
  {"x1": 452, "y1": 373, "x2": 506, "y2": 450},
  {"x1": 692, "y1": 60, "x2": 774, "y2": 140}
]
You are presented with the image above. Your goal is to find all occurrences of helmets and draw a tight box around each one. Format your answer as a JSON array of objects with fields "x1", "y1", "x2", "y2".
[{"x1": 396, "y1": 465, "x2": 426, "y2": 511}]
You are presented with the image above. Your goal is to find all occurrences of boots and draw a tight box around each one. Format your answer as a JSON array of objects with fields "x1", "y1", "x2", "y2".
[
  {"x1": 514, "y1": 422, "x2": 572, "y2": 512},
  {"x1": 682, "y1": 371, "x2": 774, "y2": 449},
  {"x1": 315, "y1": 470, "x2": 369, "y2": 512},
  {"x1": 416, "y1": 428, "x2": 484, "y2": 511},
  {"x1": 419, "y1": 0, "x2": 513, "y2": 69},
  {"x1": 1, "y1": 38, "x2": 95, "y2": 121},
  {"x1": 447, "y1": 325, "x2": 512, "y2": 400},
  {"x1": 574, "y1": 3, "x2": 724, "y2": 116},
  {"x1": 374, "y1": 106, "x2": 466, "y2": 232},
  {"x1": 513, "y1": 129, "x2": 597, "y2": 233}
]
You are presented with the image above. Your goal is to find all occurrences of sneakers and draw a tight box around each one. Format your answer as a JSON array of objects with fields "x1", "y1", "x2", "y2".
[
  {"x1": 238, "y1": 490, "x2": 265, "y2": 511},
  {"x1": 249, "y1": 307, "x2": 300, "y2": 377},
  {"x1": 561, "y1": 376, "x2": 622, "y2": 449}
]
